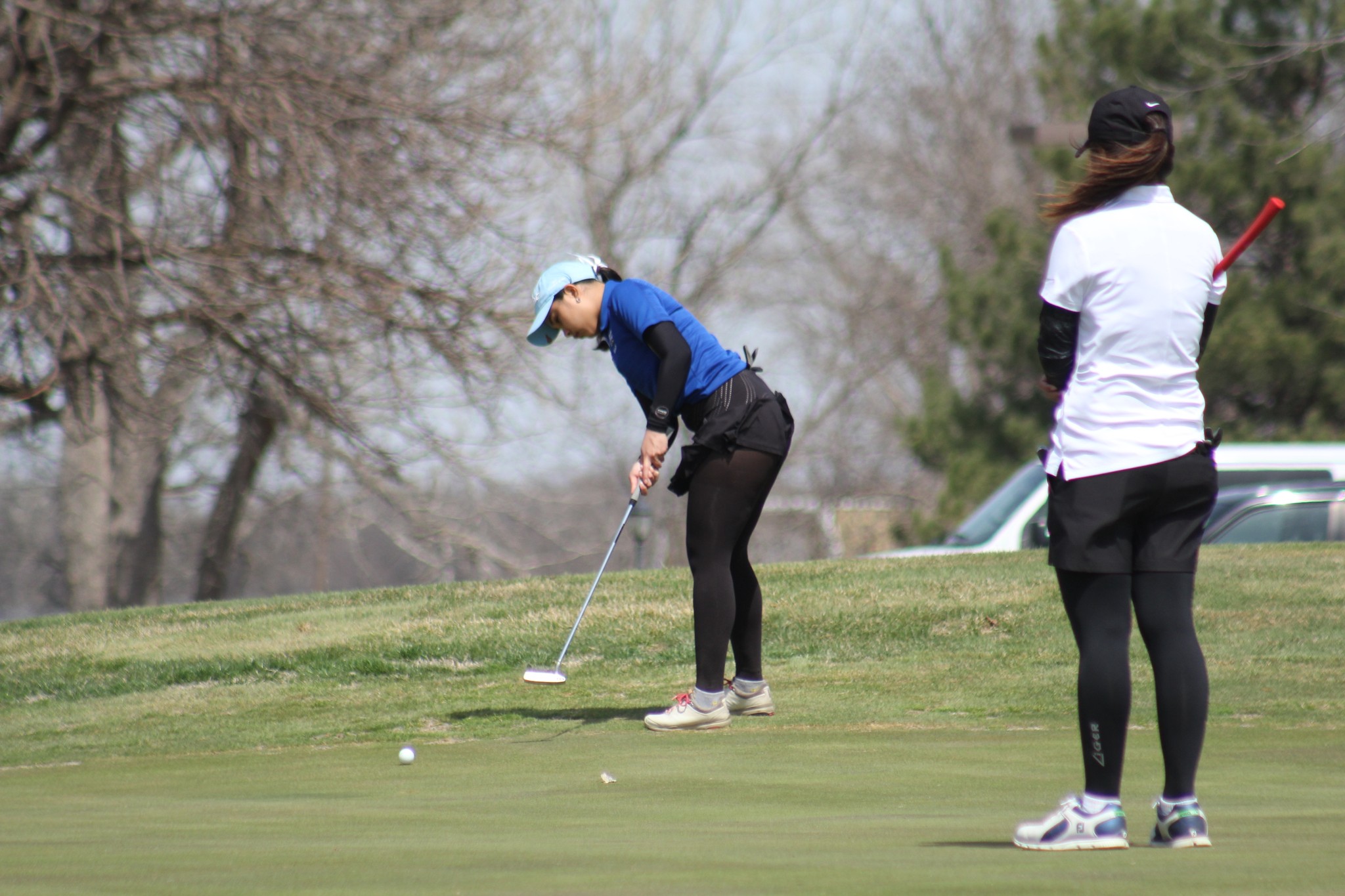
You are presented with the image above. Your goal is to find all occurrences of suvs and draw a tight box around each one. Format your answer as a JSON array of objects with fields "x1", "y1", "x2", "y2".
[{"x1": 865, "y1": 441, "x2": 1345, "y2": 557}]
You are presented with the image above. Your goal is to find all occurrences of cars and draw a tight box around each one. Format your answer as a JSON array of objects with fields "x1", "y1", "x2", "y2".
[{"x1": 1202, "y1": 480, "x2": 1345, "y2": 545}]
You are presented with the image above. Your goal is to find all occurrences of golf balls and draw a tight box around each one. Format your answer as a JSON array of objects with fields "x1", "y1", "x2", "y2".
[{"x1": 399, "y1": 748, "x2": 416, "y2": 764}]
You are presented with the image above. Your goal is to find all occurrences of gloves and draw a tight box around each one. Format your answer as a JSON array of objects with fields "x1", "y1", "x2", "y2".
[
  {"x1": 744, "y1": 345, "x2": 762, "y2": 372},
  {"x1": 1198, "y1": 428, "x2": 1223, "y2": 458}
]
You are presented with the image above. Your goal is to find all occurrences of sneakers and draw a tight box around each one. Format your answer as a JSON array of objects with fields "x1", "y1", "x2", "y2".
[
  {"x1": 644, "y1": 688, "x2": 731, "y2": 732},
  {"x1": 723, "y1": 677, "x2": 774, "y2": 716},
  {"x1": 1013, "y1": 796, "x2": 1130, "y2": 851},
  {"x1": 1149, "y1": 800, "x2": 1212, "y2": 848}
]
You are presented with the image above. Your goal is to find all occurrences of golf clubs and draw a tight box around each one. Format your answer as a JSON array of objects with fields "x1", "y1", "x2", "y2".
[{"x1": 520, "y1": 466, "x2": 649, "y2": 682}]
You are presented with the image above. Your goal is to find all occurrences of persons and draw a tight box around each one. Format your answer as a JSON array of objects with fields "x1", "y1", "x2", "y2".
[
  {"x1": 527, "y1": 254, "x2": 793, "y2": 731},
  {"x1": 1014, "y1": 85, "x2": 1229, "y2": 850}
]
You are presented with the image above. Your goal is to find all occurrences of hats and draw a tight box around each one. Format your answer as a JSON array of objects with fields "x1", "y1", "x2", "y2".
[
  {"x1": 1075, "y1": 85, "x2": 1172, "y2": 158},
  {"x1": 526, "y1": 261, "x2": 599, "y2": 347}
]
